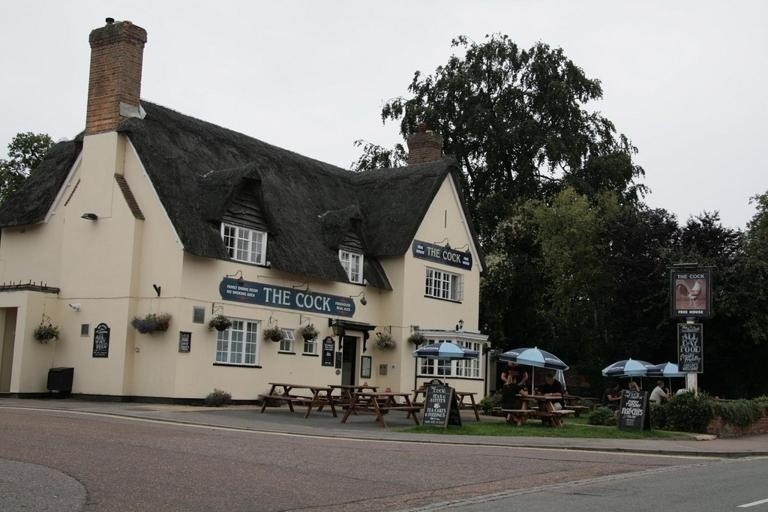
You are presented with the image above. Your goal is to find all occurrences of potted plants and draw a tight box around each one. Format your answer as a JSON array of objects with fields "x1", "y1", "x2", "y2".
[
  {"x1": 35, "y1": 325, "x2": 57, "y2": 341},
  {"x1": 209, "y1": 314, "x2": 231, "y2": 331},
  {"x1": 265, "y1": 325, "x2": 284, "y2": 342},
  {"x1": 299, "y1": 323, "x2": 318, "y2": 340}
]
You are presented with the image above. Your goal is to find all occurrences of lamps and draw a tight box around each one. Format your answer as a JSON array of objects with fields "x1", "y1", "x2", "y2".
[
  {"x1": 292, "y1": 281, "x2": 312, "y2": 295},
  {"x1": 349, "y1": 291, "x2": 368, "y2": 306},
  {"x1": 225, "y1": 270, "x2": 247, "y2": 286}
]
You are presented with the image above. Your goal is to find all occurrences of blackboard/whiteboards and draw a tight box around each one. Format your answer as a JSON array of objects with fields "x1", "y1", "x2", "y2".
[
  {"x1": 618, "y1": 390, "x2": 648, "y2": 432},
  {"x1": 422, "y1": 385, "x2": 453, "y2": 428}
]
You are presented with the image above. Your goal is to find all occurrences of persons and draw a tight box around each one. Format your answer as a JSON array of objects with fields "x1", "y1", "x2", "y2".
[
  {"x1": 500, "y1": 372, "x2": 529, "y2": 425},
  {"x1": 536, "y1": 373, "x2": 564, "y2": 426},
  {"x1": 600, "y1": 381, "x2": 621, "y2": 414},
  {"x1": 619, "y1": 381, "x2": 643, "y2": 418},
  {"x1": 648, "y1": 381, "x2": 669, "y2": 410},
  {"x1": 500, "y1": 360, "x2": 530, "y2": 425}
]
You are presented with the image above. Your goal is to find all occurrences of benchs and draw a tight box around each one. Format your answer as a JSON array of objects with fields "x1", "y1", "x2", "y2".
[
  {"x1": 257, "y1": 394, "x2": 313, "y2": 414},
  {"x1": 500, "y1": 408, "x2": 537, "y2": 424}
]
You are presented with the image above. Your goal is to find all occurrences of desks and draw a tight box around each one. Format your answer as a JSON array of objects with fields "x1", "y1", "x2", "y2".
[
  {"x1": 513, "y1": 393, "x2": 601, "y2": 429},
  {"x1": 261, "y1": 384, "x2": 481, "y2": 429}
]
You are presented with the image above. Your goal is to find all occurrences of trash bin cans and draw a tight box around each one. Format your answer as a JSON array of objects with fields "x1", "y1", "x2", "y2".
[{"x1": 47, "y1": 367, "x2": 74, "y2": 390}]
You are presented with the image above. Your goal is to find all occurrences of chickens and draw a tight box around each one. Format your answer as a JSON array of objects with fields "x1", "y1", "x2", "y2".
[{"x1": 676, "y1": 279, "x2": 704, "y2": 306}]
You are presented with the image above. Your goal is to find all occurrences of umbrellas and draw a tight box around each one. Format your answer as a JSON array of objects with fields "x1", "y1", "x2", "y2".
[
  {"x1": 600, "y1": 357, "x2": 662, "y2": 378},
  {"x1": 656, "y1": 361, "x2": 684, "y2": 378},
  {"x1": 498, "y1": 345, "x2": 569, "y2": 396},
  {"x1": 411, "y1": 339, "x2": 479, "y2": 386}
]
